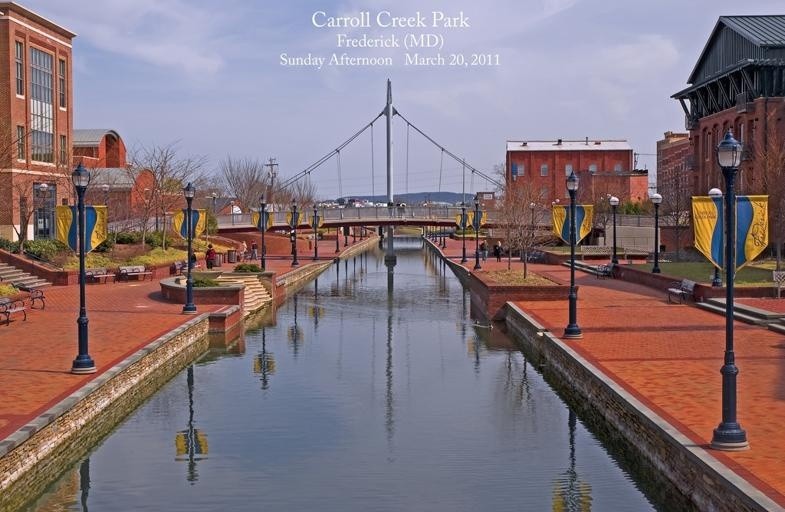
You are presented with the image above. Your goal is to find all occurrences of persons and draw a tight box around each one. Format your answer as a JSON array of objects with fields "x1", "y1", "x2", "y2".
[
  {"x1": 250, "y1": 239, "x2": 258, "y2": 261},
  {"x1": 239, "y1": 240, "x2": 247, "y2": 261},
  {"x1": 206, "y1": 244, "x2": 216, "y2": 269},
  {"x1": 495, "y1": 240, "x2": 503, "y2": 262},
  {"x1": 480, "y1": 239, "x2": 488, "y2": 261},
  {"x1": 192, "y1": 248, "x2": 196, "y2": 266}
]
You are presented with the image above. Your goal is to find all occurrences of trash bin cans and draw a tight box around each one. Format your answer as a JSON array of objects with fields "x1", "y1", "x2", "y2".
[
  {"x1": 212, "y1": 253, "x2": 223, "y2": 266},
  {"x1": 228, "y1": 250, "x2": 237, "y2": 263},
  {"x1": 660, "y1": 245, "x2": 665, "y2": 253}
]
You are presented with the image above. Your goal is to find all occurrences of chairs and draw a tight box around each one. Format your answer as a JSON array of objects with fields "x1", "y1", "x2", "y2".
[
  {"x1": 0, "y1": 299, "x2": 27, "y2": 326},
  {"x1": 86, "y1": 269, "x2": 116, "y2": 284},
  {"x1": 667, "y1": 278, "x2": 696, "y2": 304},
  {"x1": 174, "y1": 260, "x2": 188, "y2": 276},
  {"x1": 597, "y1": 261, "x2": 615, "y2": 281},
  {"x1": 118, "y1": 266, "x2": 153, "y2": 283},
  {"x1": 10, "y1": 280, "x2": 46, "y2": 310}
]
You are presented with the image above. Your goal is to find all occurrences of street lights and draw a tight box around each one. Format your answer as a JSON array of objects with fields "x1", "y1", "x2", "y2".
[
  {"x1": 70, "y1": 161, "x2": 97, "y2": 375},
  {"x1": 460, "y1": 195, "x2": 481, "y2": 269},
  {"x1": 212, "y1": 192, "x2": 216, "y2": 214},
  {"x1": 231, "y1": 200, "x2": 234, "y2": 214},
  {"x1": 183, "y1": 181, "x2": 197, "y2": 315},
  {"x1": 551, "y1": 198, "x2": 560, "y2": 207},
  {"x1": 709, "y1": 127, "x2": 750, "y2": 450},
  {"x1": 707, "y1": 188, "x2": 723, "y2": 287},
  {"x1": 529, "y1": 202, "x2": 537, "y2": 246},
  {"x1": 610, "y1": 196, "x2": 620, "y2": 264},
  {"x1": 259, "y1": 193, "x2": 318, "y2": 271},
  {"x1": 651, "y1": 193, "x2": 663, "y2": 273},
  {"x1": 562, "y1": 170, "x2": 582, "y2": 339}
]
